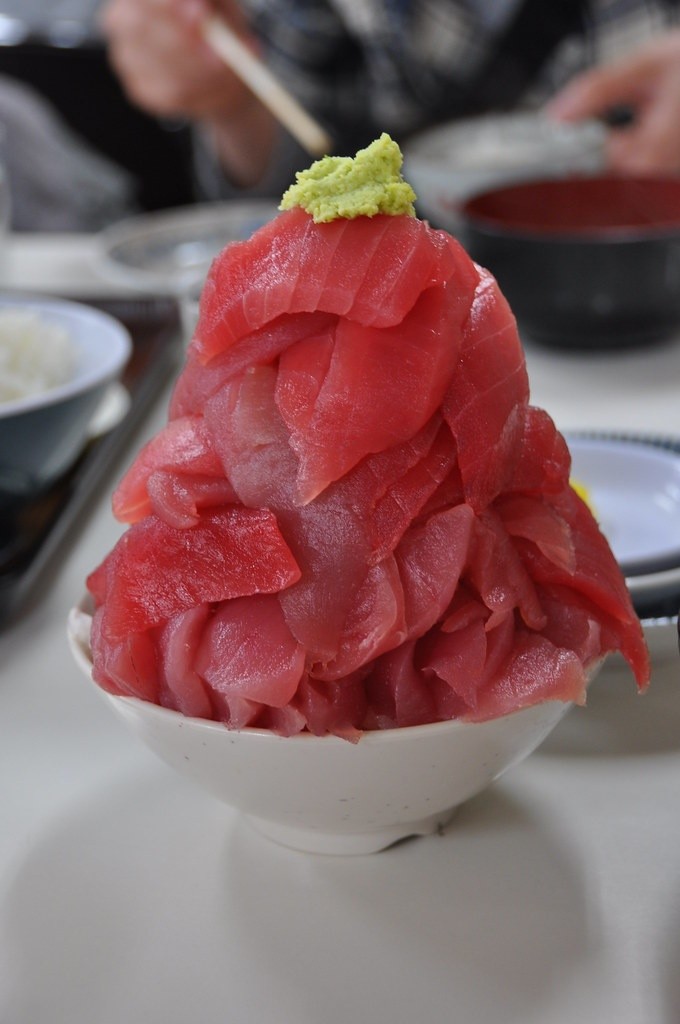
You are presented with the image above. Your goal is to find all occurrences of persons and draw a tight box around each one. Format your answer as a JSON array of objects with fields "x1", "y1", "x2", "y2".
[{"x1": 94, "y1": 1, "x2": 680, "y2": 235}]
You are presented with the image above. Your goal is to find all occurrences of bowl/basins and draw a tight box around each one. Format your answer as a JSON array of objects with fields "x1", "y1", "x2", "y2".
[
  {"x1": 68, "y1": 592, "x2": 608, "y2": 855},
  {"x1": 560, "y1": 427, "x2": 680, "y2": 666},
  {"x1": 0, "y1": 294, "x2": 133, "y2": 494},
  {"x1": 454, "y1": 173, "x2": 680, "y2": 355},
  {"x1": 92, "y1": 201, "x2": 281, "y2": 365},
  {"x1": 403, "y1": 111, "x2": 609, "y2": 229}
]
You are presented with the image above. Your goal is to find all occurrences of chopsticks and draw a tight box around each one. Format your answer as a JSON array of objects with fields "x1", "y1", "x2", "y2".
[{"x1": 204, "y1": 16, "x2": 331, "y2": 153}]
